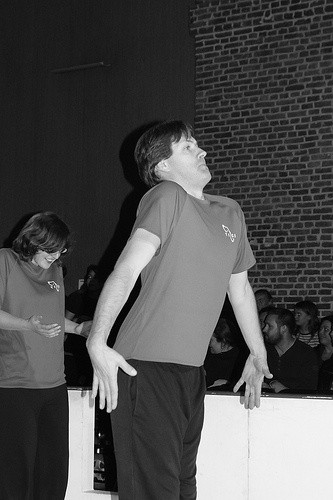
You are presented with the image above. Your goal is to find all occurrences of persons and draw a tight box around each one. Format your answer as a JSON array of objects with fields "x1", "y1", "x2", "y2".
[
  {"x1": 201, "y1": 288, "x2": 333, "y2": 398},
  {"x1": 59, "y1": 262, "x2": 143, "y2": 388},
  {"x1": 85, "y1": 121, "x2": 274, "y2": 500},
  {"x1": 0, "y1": 210, "x2": 93, "y2": 500}
]
